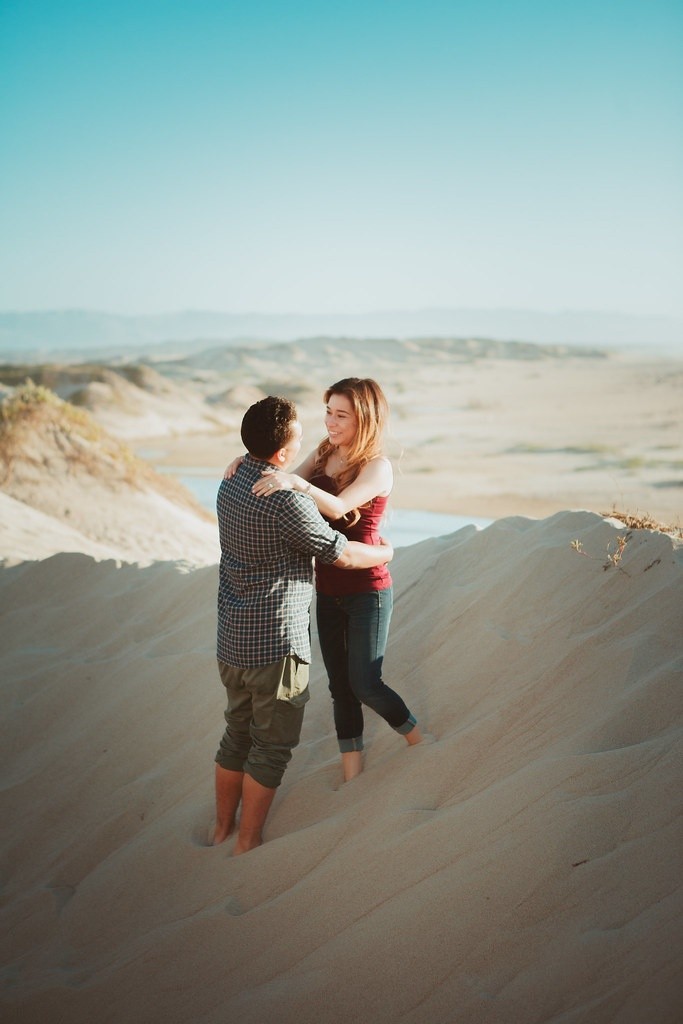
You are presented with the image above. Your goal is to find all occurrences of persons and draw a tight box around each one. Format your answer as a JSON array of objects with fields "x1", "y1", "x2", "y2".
[
  {"x1": 224, "y1": 377, "x2": 422, "y2": 783},
  {"x1": 210, "y1": 395, "x2": 393, "y2": 857}
]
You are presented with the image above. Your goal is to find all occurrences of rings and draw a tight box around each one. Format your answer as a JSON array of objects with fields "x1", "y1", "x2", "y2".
[{"x1": 267, "y1": 482, "x2": 273, "y2": 489}]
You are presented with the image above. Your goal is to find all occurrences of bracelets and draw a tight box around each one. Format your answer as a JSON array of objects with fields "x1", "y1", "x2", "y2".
[{"x1": 306, "y1": 483, "x2": 312, "y2": 496}]
